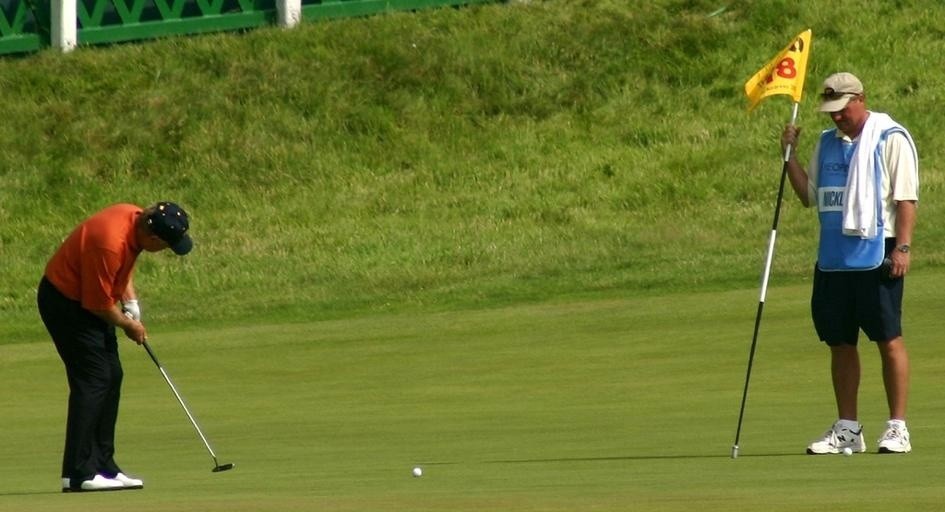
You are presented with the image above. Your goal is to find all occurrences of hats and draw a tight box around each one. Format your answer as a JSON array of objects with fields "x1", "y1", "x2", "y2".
[
  {"x1": 146, "y1": 201, "x2": 192, "y2": 256},
  {"x1": 818, "y1": 73, "x2": 864, "y2": 114}
]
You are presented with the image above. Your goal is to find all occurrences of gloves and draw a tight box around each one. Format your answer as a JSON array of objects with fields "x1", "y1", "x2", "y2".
[{"x1": 122, "y1": 299, "x2": 140, "y2": 325}]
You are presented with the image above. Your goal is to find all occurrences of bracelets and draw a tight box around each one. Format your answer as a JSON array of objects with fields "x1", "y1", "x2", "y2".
[{"x1": 895, "y1": 243, "x2": 911, "y2": 253}]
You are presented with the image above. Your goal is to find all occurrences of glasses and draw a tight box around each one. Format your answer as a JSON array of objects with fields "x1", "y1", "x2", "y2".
[{"x1": 817, "y1": 88, "x2": 857, "y2": 99}]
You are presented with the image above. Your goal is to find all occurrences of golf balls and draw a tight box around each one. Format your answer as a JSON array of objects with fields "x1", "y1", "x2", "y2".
[
  {"x1": 413, "y1": 468, "x2": 422, "y2": 477},
  {"x1": 841, "y1": 448, "x2": 852, "y2": 456}
]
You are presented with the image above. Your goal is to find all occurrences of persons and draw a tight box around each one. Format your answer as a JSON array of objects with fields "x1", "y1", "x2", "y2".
[
  {"x1": 37, "y1": 197, "x2": 194, "y2": 493},
  {"x1": 781, "y1": 71, "x2": 920, "y2": 455}
]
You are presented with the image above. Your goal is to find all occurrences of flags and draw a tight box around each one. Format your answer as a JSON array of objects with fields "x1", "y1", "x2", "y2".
[{"x1": 742, "y1": 27, "x2": 812, "y2": 113}]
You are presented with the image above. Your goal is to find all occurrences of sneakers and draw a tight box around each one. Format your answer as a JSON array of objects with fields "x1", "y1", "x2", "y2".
[
  {"x1": 806, "y1": 421, "x2": 867, "y2": 454},
  {"x1": 877, "y1": 421, "x2": 912, "y2": 453},
  {"x1": 110, "y1": 473, "x2": 144, "y2": 490},
  {"x1": 61, "y1": 472, "x2": 124, "y2": 492}
]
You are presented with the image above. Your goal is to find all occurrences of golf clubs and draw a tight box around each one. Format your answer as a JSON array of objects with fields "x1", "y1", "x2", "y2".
[{"x1": 122, "y1": 308, "x2": 234, "y2": 472}]
